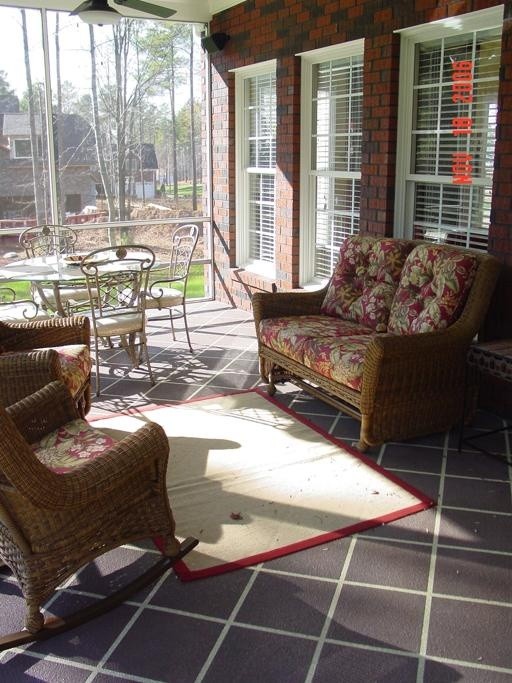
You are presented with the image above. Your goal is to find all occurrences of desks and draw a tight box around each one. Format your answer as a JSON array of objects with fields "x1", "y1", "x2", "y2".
[{"x1": 3, "y1": 250, "x2": 154, "y2": 370}]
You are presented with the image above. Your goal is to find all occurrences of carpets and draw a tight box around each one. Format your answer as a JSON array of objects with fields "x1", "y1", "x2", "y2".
[{"x1": 85, "y1": 385, "x2": 439, "y2": 583}]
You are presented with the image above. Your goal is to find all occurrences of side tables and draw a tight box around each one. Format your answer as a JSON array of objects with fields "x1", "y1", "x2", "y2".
[{"x1": 457, "y1": 338, "x2": 512, "y2": 465}]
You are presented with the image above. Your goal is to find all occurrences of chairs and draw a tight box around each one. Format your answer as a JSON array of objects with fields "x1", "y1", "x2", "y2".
[
  {"x1": 72, "y1": 244, "x2": 157, "y2": 397},
  {"x1": 1, "y1": 376, "x2": 201, "y2": 652},
  {"x1": 0, "y1": 286, "x2": 50, "y2": 323},
  {"x1": 18, "y1": 224, "x2": 101, "y2": 312},
  {"x1": 141, "y1": 224, "x2": 200, "y2": 354}
]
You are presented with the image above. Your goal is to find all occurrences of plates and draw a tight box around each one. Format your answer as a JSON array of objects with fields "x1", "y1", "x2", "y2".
[{"x1": 62, "y1": 255, "x2": 109, "y2": 265}]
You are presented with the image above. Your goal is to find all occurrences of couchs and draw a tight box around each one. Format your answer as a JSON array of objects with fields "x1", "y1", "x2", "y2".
[
  {"x1": 0, "y1": 316, "x2": 92, "y2": 430},
  {"x1": 247, "y1": 229, "x2": 503, "y2": 452}
]
